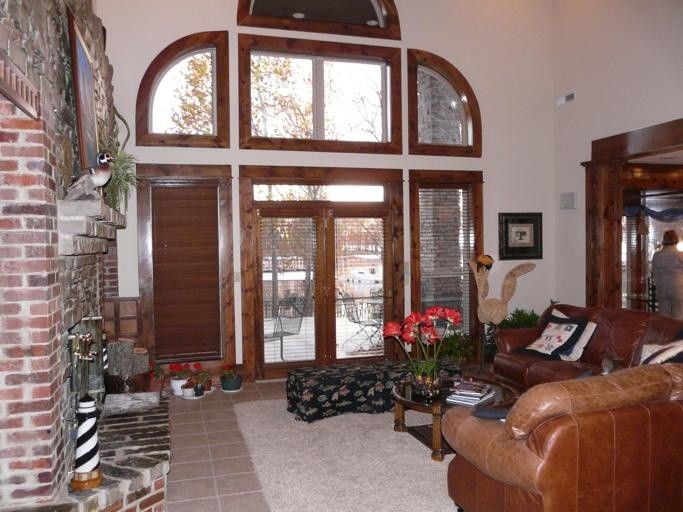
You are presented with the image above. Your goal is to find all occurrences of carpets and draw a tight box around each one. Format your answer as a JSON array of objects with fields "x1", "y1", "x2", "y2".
[{"x1": 232, "y1": 398, "x2": 463, "y2": 511}]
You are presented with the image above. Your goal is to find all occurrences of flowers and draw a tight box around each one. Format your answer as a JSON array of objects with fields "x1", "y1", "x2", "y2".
[
  {"x1": 166, "y1": 362, "x2": 242, "y2": 397},
  {"x1": 382, "y1": 304, "x2": 462, "y2": 379}
]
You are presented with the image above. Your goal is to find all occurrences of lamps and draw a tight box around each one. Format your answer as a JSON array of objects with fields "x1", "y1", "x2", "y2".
[{"x1": 69, "y1": 392, "x2": 103, "y2": 490}]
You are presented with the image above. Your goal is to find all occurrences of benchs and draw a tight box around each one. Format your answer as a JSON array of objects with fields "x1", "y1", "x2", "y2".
[{"x1": 286, "y1": 358, "x2": 451, "y2": 423}]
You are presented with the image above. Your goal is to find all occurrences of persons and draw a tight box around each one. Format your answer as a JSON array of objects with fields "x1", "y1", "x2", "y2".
[{"x1": 649, "y1": 228, "x2": 682, "y2": 321}]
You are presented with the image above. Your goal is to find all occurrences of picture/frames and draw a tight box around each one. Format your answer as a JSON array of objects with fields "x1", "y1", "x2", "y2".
[
  {"x1": 67, "y1": 12, "x2": 103, "y2": 167},
  {"x1": 497, "y1": 212, "x2": 543, "y2": 259}
]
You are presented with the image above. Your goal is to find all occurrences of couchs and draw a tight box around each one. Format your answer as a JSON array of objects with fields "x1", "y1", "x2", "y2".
[{"x1": 440, "y1": 303, "x2": 682, "y2": 511}]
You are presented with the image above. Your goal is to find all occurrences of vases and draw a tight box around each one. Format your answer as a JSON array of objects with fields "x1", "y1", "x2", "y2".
[
  {"x1": 392, "y1": 381, "x2": 449, "y2": 402},
  {"x1": 171, "y1": 377, "x2": 241, "y2": 396}
]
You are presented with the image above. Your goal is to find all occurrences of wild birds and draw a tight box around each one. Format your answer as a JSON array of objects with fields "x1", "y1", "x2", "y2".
[{"x1": 67, "y1": 151, "x2": 115, "y2": 191}]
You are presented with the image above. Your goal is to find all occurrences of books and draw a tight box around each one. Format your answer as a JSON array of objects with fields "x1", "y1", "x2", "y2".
[{"x1": 445, "y1": 379, "x2": 495, "y2": 408}]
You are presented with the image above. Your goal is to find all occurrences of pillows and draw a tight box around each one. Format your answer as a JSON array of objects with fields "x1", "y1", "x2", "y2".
[
  {"x1": 639, "y1": 341, "x2": 683, "y2": 366},
  {"x1": 514, "y1": 310, "x2": 596, "y2": 362}
]
